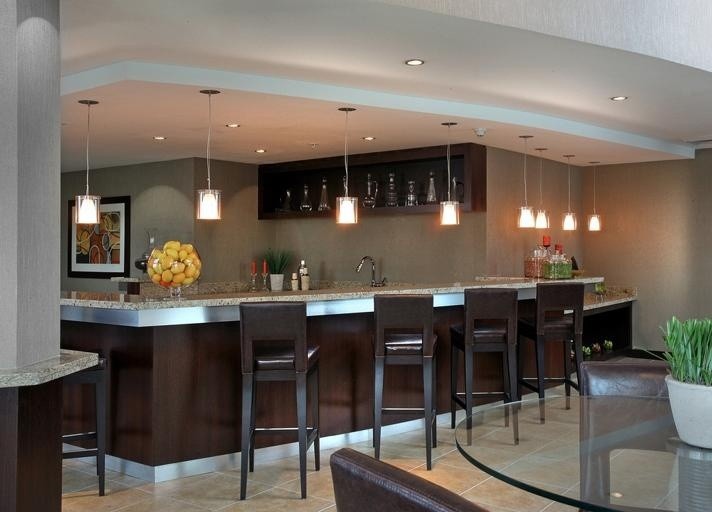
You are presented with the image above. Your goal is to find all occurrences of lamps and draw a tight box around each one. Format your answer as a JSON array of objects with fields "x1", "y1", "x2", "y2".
[
  {"x1": 75, "y1": 100, "x2": 100, "y2": 225},
  {"x1": 517, "y1": 135, "x2": 602, "y2": 231},
  {"x1": 336, "y1": 107, "x2": 358, "y2": 226},
  {"x1": 196, "y1": 89, "x2": 221, "y2": 221},
  {"x1": 439, "y1": 122, "x2": 458, "y2": 227}
]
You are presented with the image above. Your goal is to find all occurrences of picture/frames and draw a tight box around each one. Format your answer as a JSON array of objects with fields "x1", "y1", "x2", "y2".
[{"x1": 67, "y1": 196, "x2": 131, "y2": 279}]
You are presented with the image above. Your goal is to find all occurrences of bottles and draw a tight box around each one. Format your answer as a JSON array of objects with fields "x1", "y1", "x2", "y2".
[
  {"x1": 360, "y1": 173, "x2": 379, "y2": 209},
  {"x1": 523, "y1": 248, "x2": 552, "y2": 278},
  {"x1": 299, "y1": 184, "x2": 313, "y2": 211},
  {"x1": 290, "y1": 272, "x2": 299, "y2": 292},
  {"x1": 317, "y1": 176, "x2": 332, "y2": 212},
  {"x1": 540, "y1": 254, "x2": 574, "y2": 280},
  {"x1": 450, "y1": 176, "x2": 464, "y2": 203},
  {"x1": 341, "y1": 174, "x2": 351, "y2": 197},
  {"x1": 426, "y1": 172, "x2": 438, "y2": 204},
  {"x1": 300, "y1": 267, "x2": 311, "y2": 291},
  {"x1": 298, "y1": 259, "x2": 307, "y2": 283},
  {"x1": 283, "y1": 189, "x2": 295, "y2": 211},
  {"x1": 135, "y1": 226, "x2": 163, "y2": 280},
  {"x1": 386, "y1": 172, "x2": 400, "y2": 207},
  {"x1": 406, "y1": 178, "x2": 419, "y2": 207}
]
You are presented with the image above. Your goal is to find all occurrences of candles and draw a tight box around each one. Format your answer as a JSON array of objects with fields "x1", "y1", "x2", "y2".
[
  {"x1": 543, "y1": 234, "x2": 551, "y2": 245},
  {"x1": 555, "y1": 243, "x2": 564, "y2": 254},
  {"x1": 262, "y1": 259, "x2": 268, "y2": 273},
  {"x1": 252, "y1": 259, "x2": 257, "y2": 274}
]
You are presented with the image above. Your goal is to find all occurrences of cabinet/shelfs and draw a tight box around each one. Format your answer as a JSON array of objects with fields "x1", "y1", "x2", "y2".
[{"x1": 258, "y1": 143, "x2": 487, "y2": 220}]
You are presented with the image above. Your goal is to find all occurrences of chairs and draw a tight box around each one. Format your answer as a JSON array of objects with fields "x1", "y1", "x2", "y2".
[
  {"x1": 62, "y1": 358, "x2": 109, "y2": 495},
  {"x1": 239, "y1": 302, "x2": 321, "y2": 499},
  {"x1": 518, "y1": 282, "x2": 585, "y2": 424},
  {"x1": 450, "y1": 288, "x2": 519, "y2": 447},
  {"x1": 331, "y1": 448, "x2": 489, "y2": 512},
  {"x1": 374, "y1": 294, "x2": 439, "y2": 471},
  {"x1": 579, "y1": 361, "x2": 678, "y2": 512}
]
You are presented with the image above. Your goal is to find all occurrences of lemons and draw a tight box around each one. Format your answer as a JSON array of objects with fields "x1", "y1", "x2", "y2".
[{"x1": 146, "y1": 240, "x2": 201, "y2": 287}]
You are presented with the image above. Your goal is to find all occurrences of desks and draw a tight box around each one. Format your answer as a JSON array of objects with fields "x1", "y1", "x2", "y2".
[{"x1": 455, "y1": 394, "x2": 712, "y2": 512}]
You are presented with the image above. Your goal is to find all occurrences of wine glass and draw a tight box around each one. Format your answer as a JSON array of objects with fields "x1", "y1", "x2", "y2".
[{"x1": 147, "y1": 244, "x2": 203, "y2": 301}]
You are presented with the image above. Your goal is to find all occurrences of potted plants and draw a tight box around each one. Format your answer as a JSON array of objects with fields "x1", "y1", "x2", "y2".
[
  {"x1": 659, "y1": 315, "x2": 712, "y2": 451},
  {"x1": 261, "y1": 248, "x2": 293, "y2": 292}
]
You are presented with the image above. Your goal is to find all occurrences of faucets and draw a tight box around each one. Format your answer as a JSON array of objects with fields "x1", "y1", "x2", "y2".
[{"x1": 356, "y1": 256, "x2": 388, "y2": 287}]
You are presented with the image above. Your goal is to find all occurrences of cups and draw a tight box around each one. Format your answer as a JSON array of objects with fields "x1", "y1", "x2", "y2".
[{"x1": 595, "y1": 282, "x2": 608, "y2": 296}]
